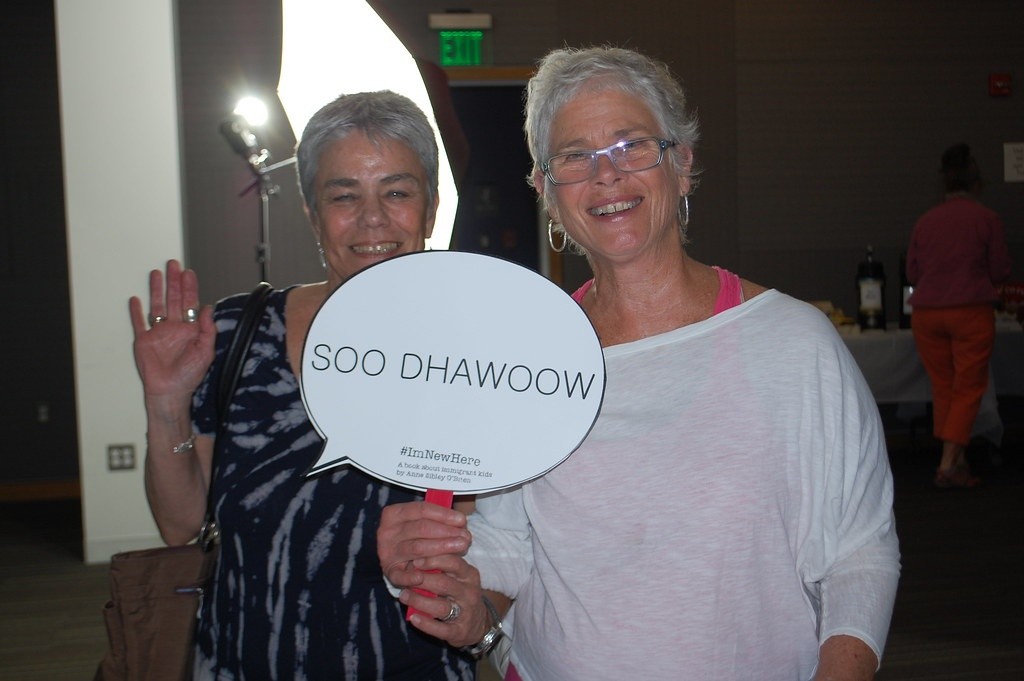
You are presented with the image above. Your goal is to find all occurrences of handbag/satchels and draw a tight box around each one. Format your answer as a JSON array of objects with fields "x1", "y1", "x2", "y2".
[{"x1": 91, "y1": 542, "x2": 216, "y2": 681}]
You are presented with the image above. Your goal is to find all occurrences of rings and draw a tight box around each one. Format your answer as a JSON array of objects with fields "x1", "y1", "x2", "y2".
[
  {"x1": 149, "y1": 315, "x2": 166, "y2": 327},
  {"x1": 182, "y1": 308, "x2": 200, "y2": 323},
  {"x1": 442, "y1": 603, "x2": 461, "y2": 622}
]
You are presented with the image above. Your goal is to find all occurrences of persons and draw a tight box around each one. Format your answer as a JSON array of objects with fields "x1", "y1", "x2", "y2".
[
  {"x1": 904, "y1": 145, "x2": 1012, "y2": 488},
  {"x1": 129, "y1": 91, "x2": 476, "y2": 680},
  {"x1": 376, "y1": 45, "x2": 903, "y2": 681}
]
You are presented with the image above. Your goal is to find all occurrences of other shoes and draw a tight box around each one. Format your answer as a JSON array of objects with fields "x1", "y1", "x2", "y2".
[{"x1": 932, "y1": 461, "x2": 980, "y2": 491}]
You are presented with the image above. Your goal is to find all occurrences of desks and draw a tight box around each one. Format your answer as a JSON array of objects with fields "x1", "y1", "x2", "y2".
[{"x1": 836, "y1": 312, "x2": 1024, "y2": 470}]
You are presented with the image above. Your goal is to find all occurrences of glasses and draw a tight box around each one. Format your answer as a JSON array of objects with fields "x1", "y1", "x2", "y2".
[{"x1": 539, "y1": 137, "x2": 680, "y2": 187}]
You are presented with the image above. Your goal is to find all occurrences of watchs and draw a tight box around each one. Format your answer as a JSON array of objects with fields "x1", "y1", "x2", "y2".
[{"x1": 464, "y1": 597, "x2": 506, "y2": 659}]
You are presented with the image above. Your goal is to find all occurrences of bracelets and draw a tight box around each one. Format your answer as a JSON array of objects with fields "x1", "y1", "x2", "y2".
[{"x1": 174, "y1": 435, "x2": 198, "y2": 456}]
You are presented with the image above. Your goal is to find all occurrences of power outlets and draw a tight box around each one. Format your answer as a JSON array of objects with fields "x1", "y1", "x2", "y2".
[{"x1": 108, "y1": 445, "x2": 134, "y2": 470}]
